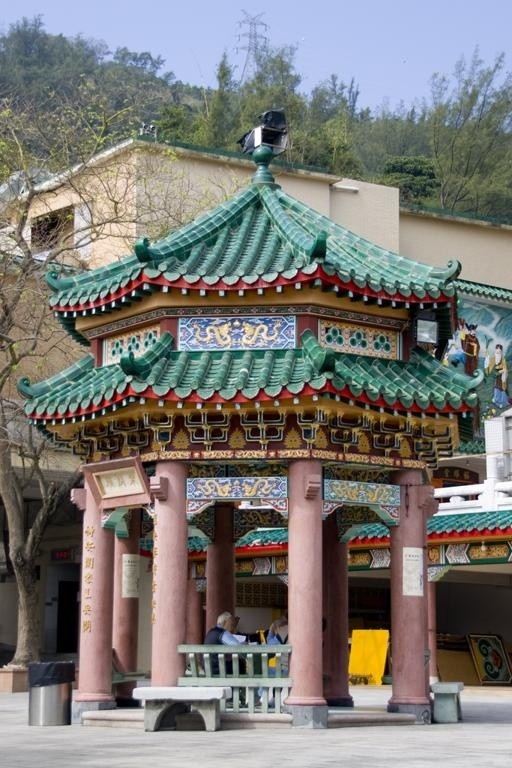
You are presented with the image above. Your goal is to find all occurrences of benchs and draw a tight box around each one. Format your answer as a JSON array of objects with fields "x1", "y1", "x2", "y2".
[
  {"x1": 132, "y1": 685, "x2": 232, "y2": 732},
  {"x1": 176, "y1": 644, "x2": 291, "y2": 715},
  {"x1": 431, "y1": 681, "x2": 464, "y2": 723},
  {"x1": 110, "y1": 649, "x2": 150, "y2": 707}
]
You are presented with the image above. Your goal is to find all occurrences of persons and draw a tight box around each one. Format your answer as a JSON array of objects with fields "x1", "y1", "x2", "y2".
[
  {"x1": 267, "y1": 609, "x2": 288, "y2": 708},
  {"x1": 202, "y1": 610, "x2": 259, "y2": 706},
  {"x1": 460, "y1": 323, "x2": 479, "y2": 374},
  {"x1": 482, "y1": 344, "x2": 511, "y2": 409}
]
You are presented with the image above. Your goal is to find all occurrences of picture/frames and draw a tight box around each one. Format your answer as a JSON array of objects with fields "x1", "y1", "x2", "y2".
[{"x1": 466, "y1": 632, "x2": 512, "y2": 686}]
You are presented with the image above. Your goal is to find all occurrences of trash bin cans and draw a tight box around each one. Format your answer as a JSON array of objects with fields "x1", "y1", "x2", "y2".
[{"x1": 28, "y1": 661, "x2": 75, "y2": 726}]
